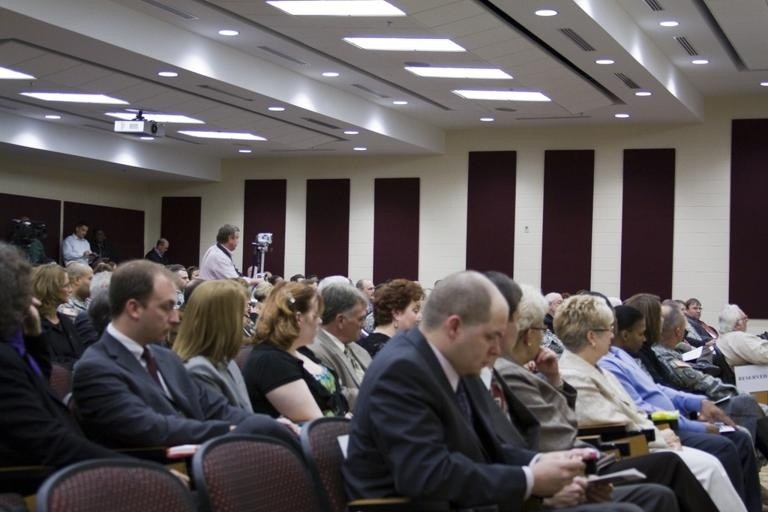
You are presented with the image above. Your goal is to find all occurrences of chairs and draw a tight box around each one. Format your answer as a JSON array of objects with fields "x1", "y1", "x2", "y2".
[
  {"x1": 192, "y1": 432, "x2": 330, "y2": 511},
  {"x1": 578, "y1": 433, "x2": 631, "y2": 461},
  {"x1": 51, "y1": 359, "x2": 70, "y2": 399},
  {"x1": 62, "y1": 389, "x2": 73, "y2": 409},
  {"x1": 300, "y1": 414, "x2": 410, "y2": 511},
  {"x1": 35, "y1": 455, "x2": 198, "y2": 512},
  {"x1": 580, "y1": 422, "x2": 629, "y2": 442}
]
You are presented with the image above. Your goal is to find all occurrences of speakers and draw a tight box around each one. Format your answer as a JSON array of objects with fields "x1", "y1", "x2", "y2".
[{"x1": 86, "y1": 218, "x2": 119, "y2": 256}]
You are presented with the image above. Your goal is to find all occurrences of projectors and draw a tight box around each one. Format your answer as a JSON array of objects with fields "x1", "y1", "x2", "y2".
[{"x1": 113, "y1": 119, "x2": 166, "y2": 141}]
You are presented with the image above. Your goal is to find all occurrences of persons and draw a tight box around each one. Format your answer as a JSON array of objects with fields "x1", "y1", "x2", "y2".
[{"x1": 0, "y1": 217, "x2": 768, "y2": 511}]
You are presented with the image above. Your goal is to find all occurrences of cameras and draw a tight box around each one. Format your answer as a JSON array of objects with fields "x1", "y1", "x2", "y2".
[{"x1": 252, "y1": 233, "x2": 274, "y2": 253}]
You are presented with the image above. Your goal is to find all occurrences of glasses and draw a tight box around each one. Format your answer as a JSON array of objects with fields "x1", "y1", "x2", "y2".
[
  {"x1": 526, "y1": 325, "x2": 546, "y2": 335},
  {"x1": 594, "y1": 325, "x2": 614, "y2": 333}
]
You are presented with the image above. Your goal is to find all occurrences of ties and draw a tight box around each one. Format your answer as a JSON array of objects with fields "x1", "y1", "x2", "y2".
[
  {"x1": 142, "y1": 349, "x2": 161, "y2": 384},
  {"x1": 343, "y1": 346, "x2": 363, "y2": 377},
  {"x1": 456, "y1": 381, "x2": 472, "y2": 423},
  {"x1": 490, "y1": 374, "x2": 509, "y2": 417}
]
